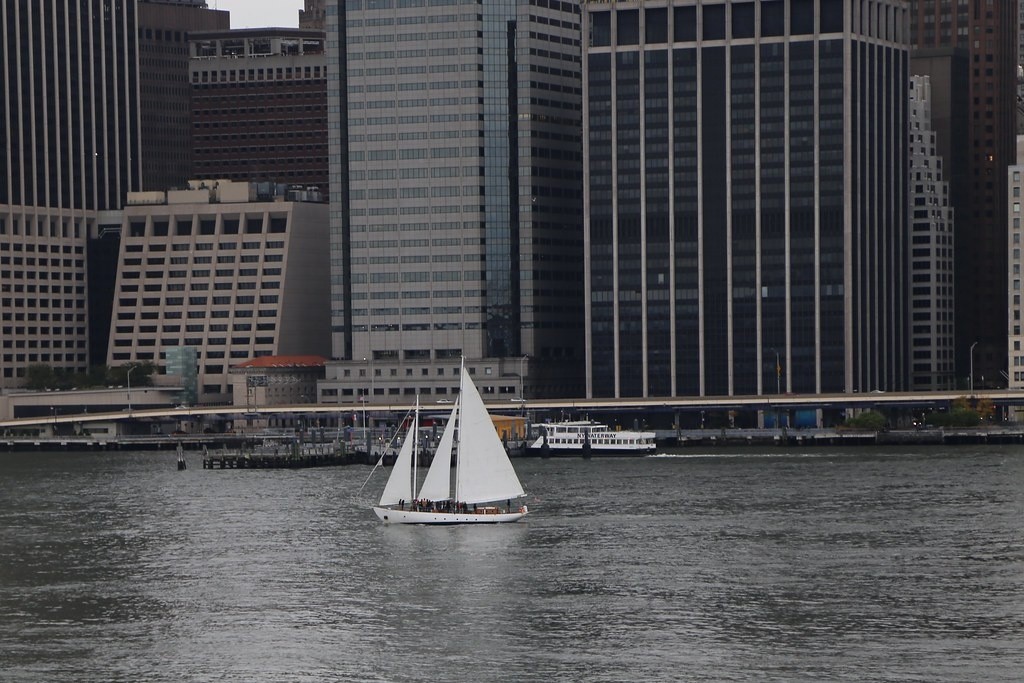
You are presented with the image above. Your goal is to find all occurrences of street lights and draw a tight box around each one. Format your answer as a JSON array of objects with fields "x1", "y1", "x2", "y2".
[
  {"x1": 771, "y1": 347, "x2": 780, "y2": 395},
  {"x1": 521, "y1": 355, "x2": 527, "y2": 406},
  {"x1": 51, "y1": 407, "x2": 57, "y2": 431},
  {"x1": 128, "y1": 366, "x2": 138, "y2": 416},
  {"x1": 364, "y1": 357, "x2": 371, "y2": 406},
  {"x1": 970, "y1": 342, "x2": 978, "y2": 395}
]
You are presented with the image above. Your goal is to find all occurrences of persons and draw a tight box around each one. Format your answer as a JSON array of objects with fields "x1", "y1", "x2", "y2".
[
  {"x1": 186, "y1": 182, "x2": 219, "y2": 190},
  {"x1": 399, "y1": 498, "x2": 477, "y2": 514}
]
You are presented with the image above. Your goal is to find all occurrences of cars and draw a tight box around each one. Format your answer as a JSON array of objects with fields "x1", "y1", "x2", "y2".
[
  {"x1": 878, "y1": 428, "x2": 891, "y2": 433},
  {"x1": 785, "y1": 393, "x2": 799, "y2": 397},
  {"x1": 871, "y1": 390, "x2": 884, "y2": 394},
  {"x1": 511, "y1": 397, "x2": 526, "y2": 402},
  {"x1": 436, "y1": 398, "x2": 455, "y2": 404},
  {"x1": 175, "y1": 405, "x2": 191, "y2": 410},
  {"x1": 168, "y1": 431, "x2": 189, "y2": 437}
]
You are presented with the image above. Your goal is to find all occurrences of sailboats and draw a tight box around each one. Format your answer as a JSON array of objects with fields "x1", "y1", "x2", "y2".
[{"x1": 348, "y1": 356, "x2": 539, "y2": 524}]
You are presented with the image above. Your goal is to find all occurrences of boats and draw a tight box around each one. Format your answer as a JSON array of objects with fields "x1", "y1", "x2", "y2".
[{"x1": 528, "y1": 421, "x2": 658, "y2": 456}]
